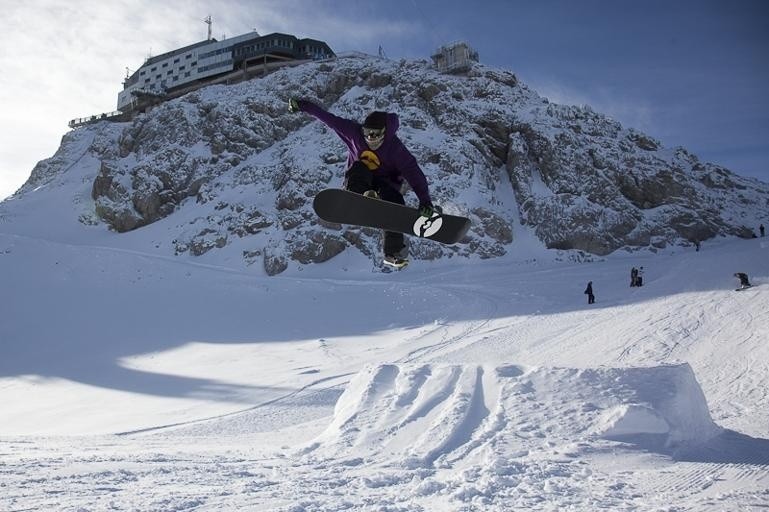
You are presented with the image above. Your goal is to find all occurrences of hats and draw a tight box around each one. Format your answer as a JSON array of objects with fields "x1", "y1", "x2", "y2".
[{"x1": 361, "y1": 110, "x2": 387, "y2": 136}]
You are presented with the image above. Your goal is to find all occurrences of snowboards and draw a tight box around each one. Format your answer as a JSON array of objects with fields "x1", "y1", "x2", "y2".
[{"x1": 313, "y1": 189, "x2": 472, "y2": 244}]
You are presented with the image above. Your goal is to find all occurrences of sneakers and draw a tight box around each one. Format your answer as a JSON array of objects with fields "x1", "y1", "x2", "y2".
[
  {"x1": 362, "y1": 189, "x2": 380, "y2": 200},
  {"x1": 383, "y1": 254, "x2": 409, "y2": 268}
]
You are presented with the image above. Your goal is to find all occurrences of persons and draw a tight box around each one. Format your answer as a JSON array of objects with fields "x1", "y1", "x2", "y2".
[
  {"x1": 585, "y1": 280, "x2": 596, "y2": 304},
  {"x1": 637, "y1": 265, "x2": 645, "y2": 286},
  {"x1": 290, "y1": 97, "x2": 433, "y2": 268},
  {"x1": 634, "y1": 267, "x2": 639, "y2": 286},
  {"x1": 630, "y1": 266, "x2": 635, "y2": 287},
  {"x1": 760, "y1": 223, "x2": 765, "y2": 237},
  {"x1": 733, "y1": 272, "x2": 750, "y2": 288}
]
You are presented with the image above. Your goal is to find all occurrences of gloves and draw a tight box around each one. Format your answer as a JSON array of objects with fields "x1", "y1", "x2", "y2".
[
  {"x1": 419, "y1": 201, "x2": 434, "y2": 218},
  {"x1": 287, "y1": 97, "x2": 301, "y2": 114}
]
